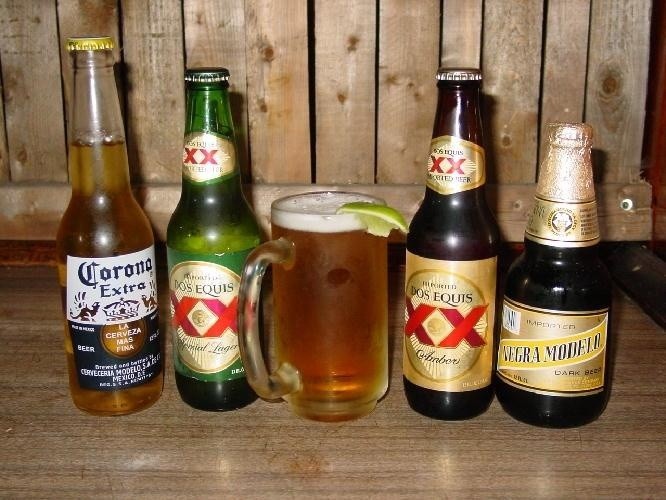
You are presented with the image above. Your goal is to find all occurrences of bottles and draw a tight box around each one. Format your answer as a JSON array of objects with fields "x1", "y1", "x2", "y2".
[
  {"x1": 164, "y1": 64, "x2": 269, "y2": 413},
  {"x1": 400, "y1": 67, "x2": 503, "y2": 422},
  {"x1": 54, "y1": 36, "x2": 165, "y2": 415},
  {"x1": 495, "y1": 121, "x2": 615, "y2": 428}
]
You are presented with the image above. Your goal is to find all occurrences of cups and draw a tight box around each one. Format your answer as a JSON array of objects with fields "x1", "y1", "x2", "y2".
[{"x1": 238, "y1": 192, "x2": 392, "y2": 420}]
